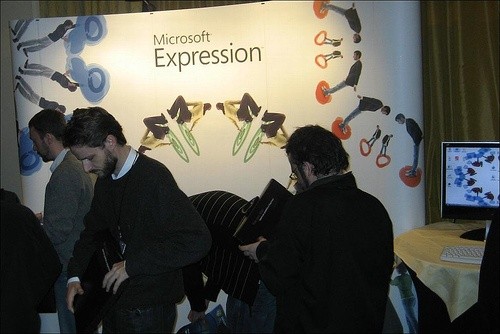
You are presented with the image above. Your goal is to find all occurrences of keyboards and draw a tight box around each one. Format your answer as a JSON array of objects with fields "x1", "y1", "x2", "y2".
[{"x1": 440, "y1": 246, "x2": 485, "y2": 264}]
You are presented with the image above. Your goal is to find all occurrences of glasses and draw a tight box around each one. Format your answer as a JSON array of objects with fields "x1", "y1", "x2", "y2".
[{"x1": 289, "y1": 166, "x2": 298, "y2": 180}]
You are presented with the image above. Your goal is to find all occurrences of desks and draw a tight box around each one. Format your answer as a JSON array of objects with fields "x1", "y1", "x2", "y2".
[{"x1": 393, "y1": 220, "x2": 492, "y2": 334}]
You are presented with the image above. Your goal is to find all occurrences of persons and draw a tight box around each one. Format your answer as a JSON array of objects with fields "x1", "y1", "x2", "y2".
[
  {"x1": 27, "y1": 108, "x2": 93, "y2": 334},
  {"x1": 0, "y1": 189, "x2": 63, "y2": 334},
  {"x1": 239, "y1": 124, "x2": 394, "y2": 334},
  {"x1": 10, "y1": 0, "x2": 424, "y2": 178},
  {"x1": 178, "y1": 189, "x2": 278, "y2": 334},
  {"x1": 66, "y1": 106, "x2": 210, "y2": 334}
]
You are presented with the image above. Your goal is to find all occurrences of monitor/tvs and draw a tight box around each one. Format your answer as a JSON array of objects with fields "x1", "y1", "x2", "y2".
[{"x1": 440, "y1": 141, "x2": 500, "y2": 242}]
